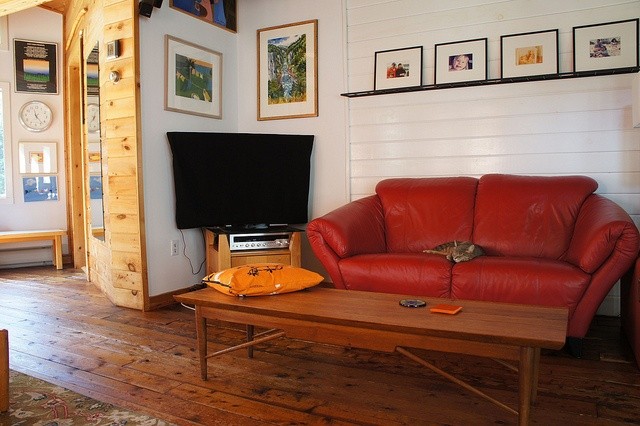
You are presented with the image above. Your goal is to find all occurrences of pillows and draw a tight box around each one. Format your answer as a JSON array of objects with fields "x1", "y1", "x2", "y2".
[{"x1": 202, "y1": 263, "x2": 322, "y2": 298}]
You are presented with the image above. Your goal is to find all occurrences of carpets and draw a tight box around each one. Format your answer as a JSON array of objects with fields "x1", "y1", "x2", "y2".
[{"x1": 0, "y1": 367, "x2": 184, "y2": 426}]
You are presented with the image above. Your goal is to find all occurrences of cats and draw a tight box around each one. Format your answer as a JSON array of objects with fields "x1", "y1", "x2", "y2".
[{"x1": 422, "y1": 239, "x2": 483, "y2": 263}]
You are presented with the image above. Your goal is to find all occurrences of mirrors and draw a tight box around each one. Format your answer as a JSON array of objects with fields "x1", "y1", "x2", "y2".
[{"x1": 86, "y1": 42, "x2": 105, "y2": 240}]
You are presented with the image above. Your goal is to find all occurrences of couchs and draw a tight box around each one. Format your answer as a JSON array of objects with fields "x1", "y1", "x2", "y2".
[
  {"x1": 307, "y1": 174, "x2": 640, "y2": 358},
  {"x1": 619, "y1": 257, "x2": 639, "y2": 372}
]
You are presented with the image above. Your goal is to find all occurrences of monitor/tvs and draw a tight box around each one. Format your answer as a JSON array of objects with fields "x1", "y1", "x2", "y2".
[{"x1": 167, "y1": 131, "x2": 315, "y2": 230}]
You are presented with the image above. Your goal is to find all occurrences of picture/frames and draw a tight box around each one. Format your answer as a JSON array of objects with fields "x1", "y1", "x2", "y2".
[
  {"x1": 88, "y1": 171, "x2": 104, "y2": 201},
  {"x1": 169, "y1": 1, "x2": 239, "y2": 34},
  {"x1": 86, "y1": 140, "x2": 103, "y2": 175},
  {"x1": 18, "y1": 174, "x2": 60, "y2": 204},
  {"x1": 19, "y1": 141, "x2": 58, "y2": 175},
  {"x1": 256, "y1": 19, "x2": 319, "y2": 120},
  {"x1": 87, "y1": 49, "x2": 98, "y2": 95},
  {"x1": 434, "y1": 38, "x2": 487, "y2": 84},
  {"x1": 106, "y1": 40, "x2": 118, "y2": 60},
  {"x1": 163, "y1": 34, "x2": 222, "y2": 119},
  {"x1": 14, "y1": 38, "x2": 58, "y2": 95},
  {"x1": 572, "y1": 19, "x2": 639, "y2": 72},
  {"x1": 374, "y1": 46, "x2": 421, "y2": 90},
  {"x1": 500, "y1": 29, "x2": 559, "y2": 78}
]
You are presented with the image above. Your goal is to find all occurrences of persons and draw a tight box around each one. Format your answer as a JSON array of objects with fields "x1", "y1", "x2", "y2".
[
  {"x1": 593, "y1": 39, "x2": 602, "y2": 57},
  {"x1": 398, "y1": 63, "x2": 406, "y2": 77},
  {"x1": 449, "y1": 54, "x2": 469, "y2": 70},
  {"x1": 388, "y1": 63, "x2": 397, "y2": 78},
  {"x1": 594, "y1": 45, "x2": 608, "y2": 56}
]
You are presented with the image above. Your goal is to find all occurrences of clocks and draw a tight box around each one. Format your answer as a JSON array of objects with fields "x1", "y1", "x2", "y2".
[
  {"x1": 18, "y1": 101, "x2": 55, "y2": 133},
  {"x1": 86, "y1": 104, "x2": 101, "y2": 133}
]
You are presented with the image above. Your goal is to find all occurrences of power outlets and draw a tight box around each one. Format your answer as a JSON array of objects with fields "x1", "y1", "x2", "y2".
[{"x1": 171, "y1": 241, "x2": 181, "y2": 257}]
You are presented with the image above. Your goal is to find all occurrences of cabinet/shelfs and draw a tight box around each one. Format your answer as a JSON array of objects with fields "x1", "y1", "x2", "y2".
[
  {"x1": 205, "y1": 232, "x2": 302, "y2": 280},
  {"x1": 341, "y1": 66, "x2": 640, "y2": 99}
]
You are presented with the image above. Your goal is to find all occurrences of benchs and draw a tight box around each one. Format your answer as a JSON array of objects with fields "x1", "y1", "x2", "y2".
[{"x1": 0, "y1": 230, "x2": 68, "y2": 269}]
